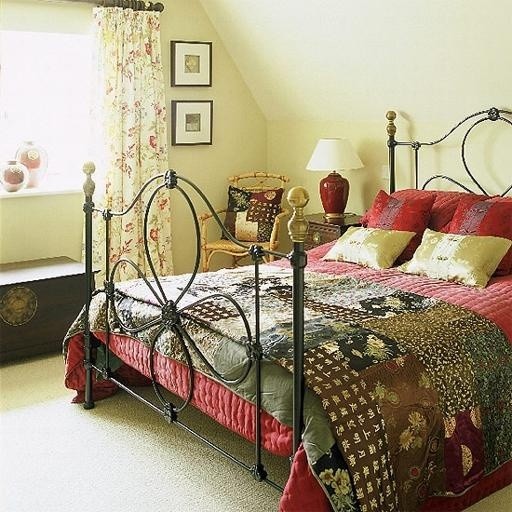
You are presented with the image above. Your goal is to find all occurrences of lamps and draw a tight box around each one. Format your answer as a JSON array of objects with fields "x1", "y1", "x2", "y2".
[{"x1": 306, "y1": 137, "x2": 365, "y2": 213}]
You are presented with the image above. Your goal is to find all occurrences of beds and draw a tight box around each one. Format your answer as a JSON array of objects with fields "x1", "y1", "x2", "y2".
[{"x1": 83, "y1": 108, "x2": 512, "y2": 512}]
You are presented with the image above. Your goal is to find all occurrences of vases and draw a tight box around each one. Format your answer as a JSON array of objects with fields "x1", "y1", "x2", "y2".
[
  {"x1": 0, "y1": 161, "x2": 29, "y2": 193},
  {"x1": 13, "y1": 140, "x2": 49, "y2": 188}
]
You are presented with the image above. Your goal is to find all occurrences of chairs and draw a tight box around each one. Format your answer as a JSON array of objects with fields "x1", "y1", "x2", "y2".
[{"x1": 199, "y1": 172, "x2": 290, "y2": 273}]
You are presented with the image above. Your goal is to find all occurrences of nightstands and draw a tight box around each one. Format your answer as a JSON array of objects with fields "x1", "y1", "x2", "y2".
[{"x1": 303, "y1": 213, "x2": 364, "y2": 251}]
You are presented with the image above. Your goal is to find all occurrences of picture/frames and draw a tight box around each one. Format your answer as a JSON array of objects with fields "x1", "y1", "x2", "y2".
[
  {"x1": 171, "y1": 40, "x2": 212, "y2": 86},
  {"x1": 171, "y1": 100, "x2": 213, "y2": 145}
]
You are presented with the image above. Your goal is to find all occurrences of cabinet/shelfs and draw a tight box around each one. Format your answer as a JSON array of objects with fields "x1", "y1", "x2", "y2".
[{"x1": 0, "y1": 256, "x2": 101, "y2": 365}]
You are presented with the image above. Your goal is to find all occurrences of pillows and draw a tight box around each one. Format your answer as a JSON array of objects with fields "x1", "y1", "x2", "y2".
[
  {"x1": 397, "y1": 227, "x2": 512, "y2": 289},
  {"x1": 450, "y1": 197, "x2": 512, "y2": 277},
  {"x1": 221, "y1": 185, "x2": 285, "y2": 243},
  {"x1": 360, "y1": 190, "x2": 482, "y2": 231},
  {"x1": 370, "y1": 190, "x2": 437, "y2": 262},
  {"x1": 320, "y1": 226, "x2": 417, "y2": 271}
]
